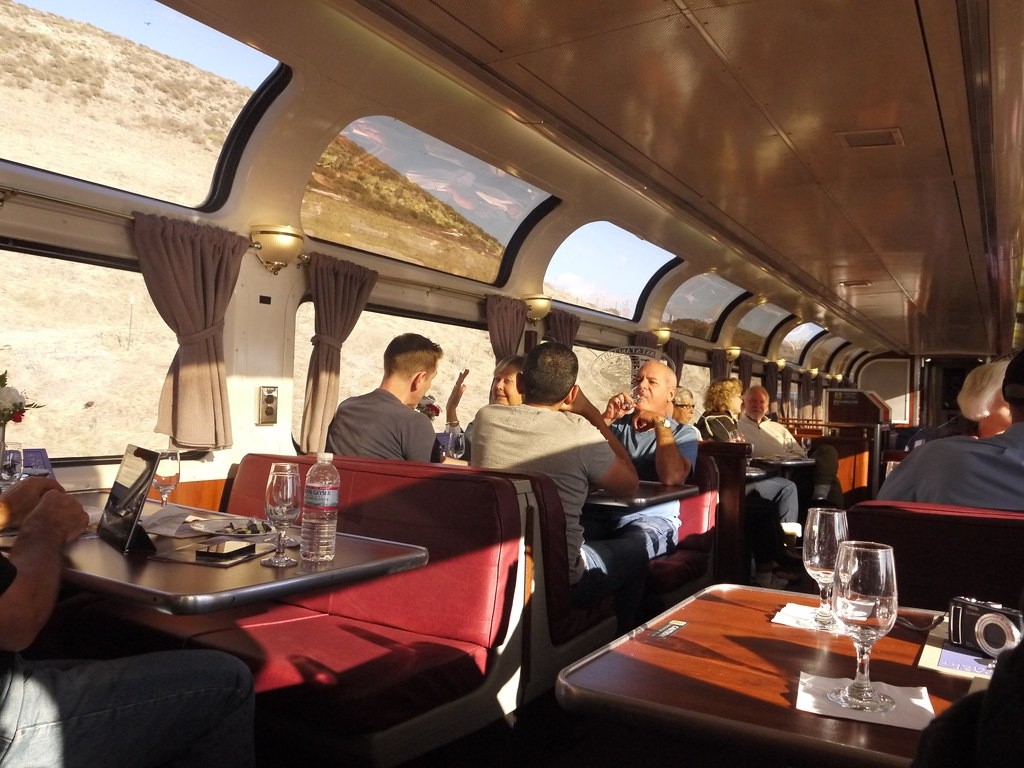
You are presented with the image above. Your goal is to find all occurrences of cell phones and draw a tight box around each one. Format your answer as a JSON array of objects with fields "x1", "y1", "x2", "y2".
[{"x1": 196, "y1": 540, "x2": 256, "y2": 559}]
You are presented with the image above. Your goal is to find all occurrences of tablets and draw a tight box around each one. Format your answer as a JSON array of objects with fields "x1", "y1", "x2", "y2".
[{"x1": 96, "y1": 444, "x2": 160, "y2": 555}]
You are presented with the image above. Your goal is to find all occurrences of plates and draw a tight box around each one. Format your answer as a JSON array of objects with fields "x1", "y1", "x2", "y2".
[{"x1": 190, "y1": 517, "x2": 276, "y2": 537}]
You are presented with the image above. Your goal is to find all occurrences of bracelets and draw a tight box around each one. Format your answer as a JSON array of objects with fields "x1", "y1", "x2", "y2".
[{"x1": 445, "y1": 421, "x2": 459, "y2": 425}]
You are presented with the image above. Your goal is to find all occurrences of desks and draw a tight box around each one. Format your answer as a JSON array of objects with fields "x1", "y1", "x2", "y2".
[
  {"x1": 584, "y1": 477, "x2": 699, "y2": 535},
  {"x1": 752, "y1": 456, "x2": 816, "y2": 480},
  {"x1": 0, "y1": 490, "x2": 429, "y2": 658},
  {"x1": 555, "y1": 582, "x2": 998, "y2": 768}
]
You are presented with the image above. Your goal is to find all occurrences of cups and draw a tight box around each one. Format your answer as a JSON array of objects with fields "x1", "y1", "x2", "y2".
[
  {"x1": 448, "y1": 432, "x2": 465, "y2": 459},
  {"x1": 0, "y1": 441, "x2": 23, "y2": 492}
]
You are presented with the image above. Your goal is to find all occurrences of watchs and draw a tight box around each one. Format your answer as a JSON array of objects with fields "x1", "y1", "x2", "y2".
[{"x1": 655, "y1": 420, "x2": 671, "y2": 428}]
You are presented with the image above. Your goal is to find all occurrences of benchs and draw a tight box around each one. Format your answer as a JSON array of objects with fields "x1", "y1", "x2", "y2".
[{"x1": 80, "y1": 420, "x2": 1024, "y2": 748}]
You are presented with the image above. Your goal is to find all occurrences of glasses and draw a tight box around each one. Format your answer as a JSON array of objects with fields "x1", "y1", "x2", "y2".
[{"x1": 673, "y1": 404, "x2": 694, "y2": 411}]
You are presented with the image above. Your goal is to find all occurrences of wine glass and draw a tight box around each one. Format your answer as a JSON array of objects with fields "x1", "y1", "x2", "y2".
[
  {"x1": 261, "y1": 462, "x2": 301, "y2": 568},
  {"x1": 783, "y1": 437, "x2": 793, "y2": 461},
  {"x1": 151, "y1": 448, "x2": 180, "y2": 507},
  {"x1": 796, "y1": 507, "x2": 849, "y2": 632},
  {"x1": 827, "y1": 540, "x2": 899, "y2": 713},
  {"x1": 801, "y1": 437, "x2": 811, "y2": 461}
]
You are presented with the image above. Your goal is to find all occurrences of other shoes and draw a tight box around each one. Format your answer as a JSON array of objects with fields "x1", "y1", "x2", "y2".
[
  {"x1": 774, "y1": 569, "x2": 801, "y2": 585},
  {"x1": 785, "y1": 548, "x2": 803, "y2": 564},
  {"x1": 755, "y1": 575, "x2": 788, "y2": 590}
]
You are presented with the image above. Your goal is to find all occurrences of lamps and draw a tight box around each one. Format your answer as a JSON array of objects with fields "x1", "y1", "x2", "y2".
[
  {"x1": 724, "y1": 346, "x2": 742, "y2": 364},
  {"x1": 798, "y1": 368, "x2": 819, "y2": 380},
  {"x1": 651, "y1": 327, "x2": 671, "y2": 349},
  {"x1": 522, "y1": 294, "x2": 552, "y2": 327},
  {"x1": 252, "y1": 225, "x2": 305, "y2": 274},
  {"x1": 764, "y1": 359, "x2": 786, "y2": 375},
  {"x1": 826, "y1": 374, "x2": 843, "y2": 384}
]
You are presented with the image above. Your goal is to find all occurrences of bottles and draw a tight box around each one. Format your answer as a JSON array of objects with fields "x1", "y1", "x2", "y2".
[{"x1": 300, "y1": 452, "x2": 340, "y2": 562}]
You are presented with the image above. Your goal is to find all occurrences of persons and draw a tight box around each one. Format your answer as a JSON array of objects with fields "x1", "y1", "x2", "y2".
[
  {"x1": 0, "y1": 477, "x2": 256, "y2": 768},
  {"x1": 737, "y1": 386, "x2": 845, "y2": 511},
  {"x1": 697, "y1": 378, "x2": 798, "y2": 592},
  {"x1": 958, "y1": 360, "x2": 1013, "y2": 440},
  {"x1": 667, "y1": 386, "x2": 703, "y2": 441},
  {"x1": 326, "y1": 334, "x2": 444, "y2": 464},
  {"x1": 600, "y1": 361, "x2": 697, "y2": 562},
  {"x1": 914, "y1": 637, "x2": 1024, "y2": 768},
  {"x1": 471, "y1": 342, "x2": 649, "y2": 639},
  {"x1": 446, "y1": 355, "x2": 527, "y2": 461},
  {"x1": 875, "y1": 349, "x2": 1024, "y2": 509}
]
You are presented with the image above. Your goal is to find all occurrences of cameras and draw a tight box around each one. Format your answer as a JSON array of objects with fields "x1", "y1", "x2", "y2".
[{"x1": 949, "y1": 595, "x2": 1024, "y2": 659}]
